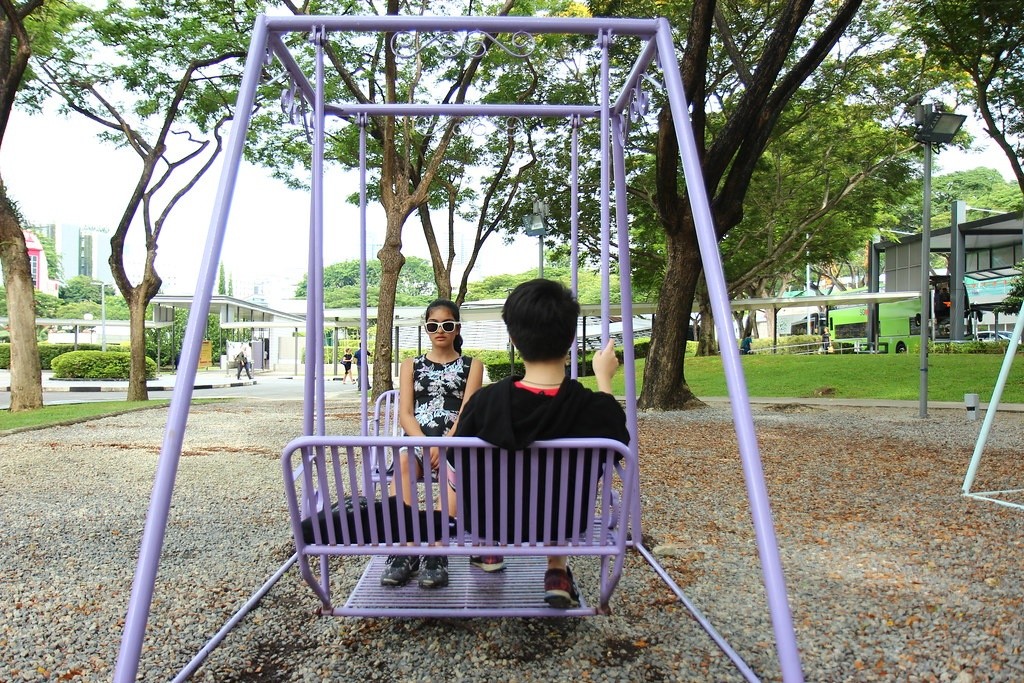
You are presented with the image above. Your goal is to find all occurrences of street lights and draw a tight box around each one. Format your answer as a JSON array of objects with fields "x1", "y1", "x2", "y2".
[
  {"x1": 520, "y1": 197, "x2": 549, "y2": 278},
  {"x1": 914, "y1": 102, "x2": 967, "y2": 421}
]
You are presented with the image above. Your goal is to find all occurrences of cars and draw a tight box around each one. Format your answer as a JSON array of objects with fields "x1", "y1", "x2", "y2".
[{"x1": 975, "y1": 328, "x2": 1021, "y2": 344}]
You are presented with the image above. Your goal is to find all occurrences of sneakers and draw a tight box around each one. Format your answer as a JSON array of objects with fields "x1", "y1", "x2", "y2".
[
  {"x1": 544, "y1": 567, "x2": 580, "y2": 605},
  {"x1": 417, "y1": 555, "x2": 449, "y2": 589},
  {"x1": 381, "y1": 555, "x2": 419, "y2": 586},
  {"x1": 469, "y1": 554, "x2": 506, "y2": 572}
]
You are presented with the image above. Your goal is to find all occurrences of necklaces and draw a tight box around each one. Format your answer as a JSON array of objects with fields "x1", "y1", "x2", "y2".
[{"x1": 521, "y1": 379, "x2": 562, "y2": 388}]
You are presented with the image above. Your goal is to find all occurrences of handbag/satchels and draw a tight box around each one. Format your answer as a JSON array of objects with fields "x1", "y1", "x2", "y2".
[
  {"x1": 291, "y1": 496, "x2": 457, "y2": 544},
  {"x1": 340, "y1": 355, "x2": 348, "y2": 365}
]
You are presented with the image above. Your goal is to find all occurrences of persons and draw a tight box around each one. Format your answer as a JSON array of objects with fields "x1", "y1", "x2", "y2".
[
  {"x1": 264, "y1": 350, "x2": 268, "y2": 368},
  {"x1": 745, "y1": 331, "x2": 752, "y2": 350},
  {"x1": 237, "y1": 343, "x2": 254, "y2": 381},
  {"x1": 822, "y1": 327, "x2": 831, "y2": 354},
  {"x1": 438, "y1": 277, "x2": 630, "y2": 609},
  {"x1": 354, "y1": 343, "x2": 373, "y2": 391},
  {"x1": 934, "y1": 286, "x2": 950, "y2": 330},
  {"x1": 379, "y1": 299, "x2": 484, "y2": 590},
  {"x1": 343, "y1": 348, "x2": 356, "y2": 384}
]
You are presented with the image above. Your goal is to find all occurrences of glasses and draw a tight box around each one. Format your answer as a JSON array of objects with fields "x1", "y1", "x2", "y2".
[{"x1": 424, "y1": 322, "x2": 461, "y2": 334}]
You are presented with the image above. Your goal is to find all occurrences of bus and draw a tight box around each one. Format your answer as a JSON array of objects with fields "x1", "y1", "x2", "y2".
[
  {"x1": 828, "y1": 283, "x2": 983, "y2": 355},
  {"x1": 790, "y1": 312, "x2": 827, "y2": 338}
]
